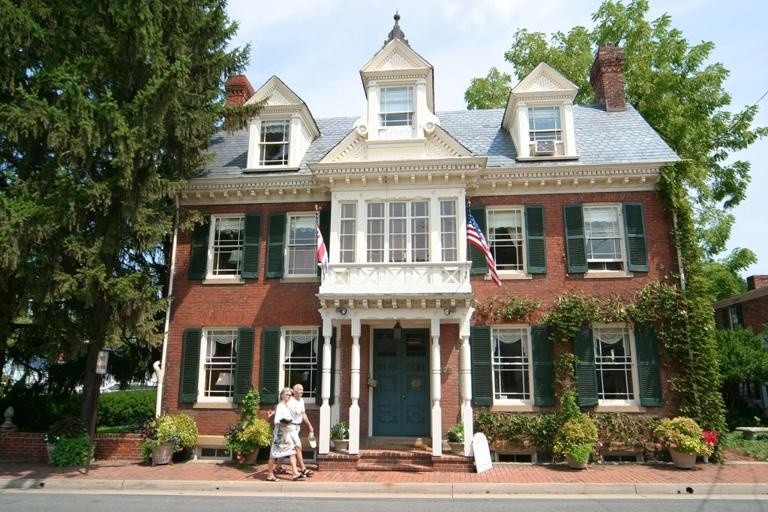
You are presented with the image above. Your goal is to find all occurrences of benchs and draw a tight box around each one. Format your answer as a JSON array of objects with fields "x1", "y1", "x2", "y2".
[{"x1": 735, "y1": 427, "x2": 768, "y2": 440}]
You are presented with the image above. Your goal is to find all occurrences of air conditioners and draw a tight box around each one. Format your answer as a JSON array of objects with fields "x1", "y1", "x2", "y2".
[{"x1": 534, "y1": 139, "x2": 557, "y2": 155}]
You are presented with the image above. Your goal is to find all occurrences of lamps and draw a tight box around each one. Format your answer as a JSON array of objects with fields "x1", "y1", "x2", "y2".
[
  {"x1": 443, "y1": 304, "x2": 457, "y2": 316},
  {"x1": 336, "y1": 307, "x2": 347, "y2": 315}
]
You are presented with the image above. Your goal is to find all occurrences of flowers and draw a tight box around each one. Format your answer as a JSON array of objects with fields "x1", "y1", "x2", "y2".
[{"x1": 702, "y1": 430, "x2": 719, "y2": 446}]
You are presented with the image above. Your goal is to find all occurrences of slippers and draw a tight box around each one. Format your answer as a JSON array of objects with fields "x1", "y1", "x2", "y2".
[
  {"x1": 266, "y1": 475, "x2": 276, "y2": 481},
  {"x1": 292, "y1": 474, "x2": 305, "y2": 481}
]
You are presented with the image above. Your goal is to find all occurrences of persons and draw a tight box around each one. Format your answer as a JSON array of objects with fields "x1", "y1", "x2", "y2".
[{"x1": 266, "y1": 384, "x2": 315, "y2": 480}]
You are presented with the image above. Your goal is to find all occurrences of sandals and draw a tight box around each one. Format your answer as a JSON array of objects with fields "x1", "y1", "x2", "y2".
[
  {"x1": 301, "y1": 469, "x2": 314, "y2": 475},
  {"x1": 274, "y1": 465, "x2": 287, "y2": 474}
]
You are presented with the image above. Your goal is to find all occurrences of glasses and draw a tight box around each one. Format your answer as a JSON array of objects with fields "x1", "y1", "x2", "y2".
[{"x1": 285, "y1": 394, "x2": 291, "y2": 397}]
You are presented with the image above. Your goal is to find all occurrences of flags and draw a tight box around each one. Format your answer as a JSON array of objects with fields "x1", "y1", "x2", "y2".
[
  {"x1": 467, "y1": 203, "x2": 503, "y2": 288},
  {"x1": 314, "y1": 212, "x2": 329, "y2": 280}
]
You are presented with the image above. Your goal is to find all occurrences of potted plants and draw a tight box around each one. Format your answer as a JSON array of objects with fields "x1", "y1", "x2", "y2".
[
  {"x1": 447, "y1": 420, "x2": 465, "y2": 453},
  {"x1": 330, "y1": 422, "x2": 350, "y2": 451},
  {"x1": 552, "y1": 389, "x2": 598, "y2": 468},
  {"x1": 223, "y1": 385, "x2": 273, "y2": 466},
  {"x1": 44, "y1": 415, "x2": 96, "y2": 469},
  {"x1": 654, "y1": 415, "x2": 714, "y2": 469},
  {"x1": 140, "y1": 413, "x2": 199, "y2": 464}
]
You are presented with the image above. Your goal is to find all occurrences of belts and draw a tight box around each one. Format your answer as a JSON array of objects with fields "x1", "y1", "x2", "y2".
[{"x1": 291, "y1": 423, "x2": 302, "y2": 425}]
